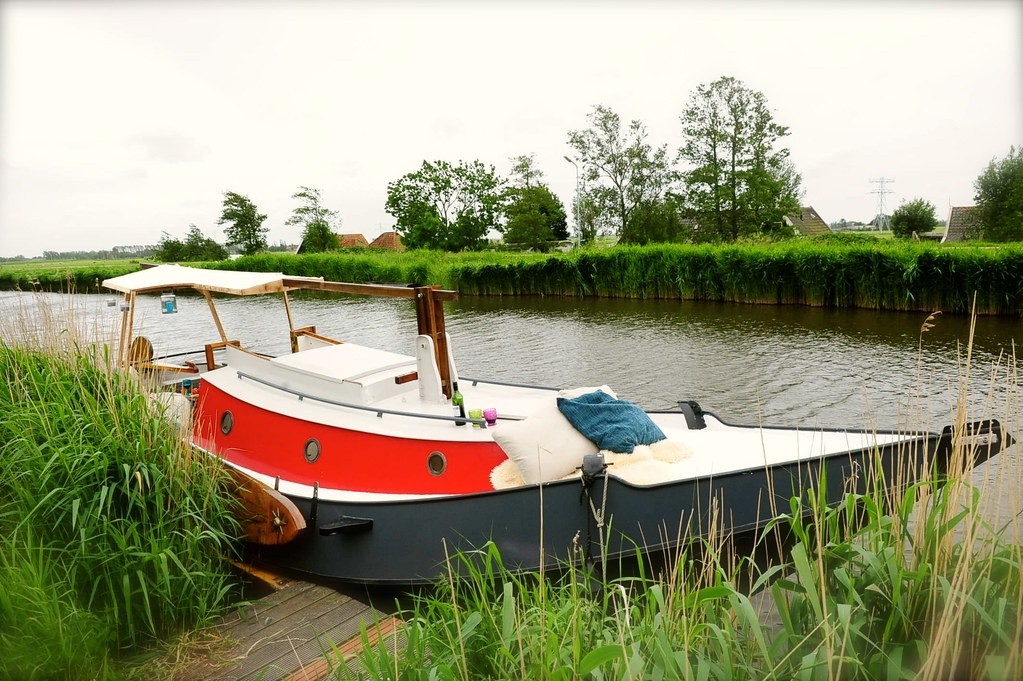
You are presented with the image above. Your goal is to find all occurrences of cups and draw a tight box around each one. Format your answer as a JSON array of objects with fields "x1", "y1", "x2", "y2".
[
  {"x1": 469, "y1": 409, "x2": 483, "y2": 428},
  {"x1": 483, "y1": 408, "x2": 497, "y2": 426}
]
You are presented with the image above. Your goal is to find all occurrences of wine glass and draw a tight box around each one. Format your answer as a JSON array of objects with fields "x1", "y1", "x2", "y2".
[{"x1": 182, "y1": 380, "x2": 191, "y2": 396}]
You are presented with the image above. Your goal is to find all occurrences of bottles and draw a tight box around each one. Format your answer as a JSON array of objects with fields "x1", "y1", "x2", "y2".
[{"x1": 452, "y1": 381, "x2": 466, "y2": 426}]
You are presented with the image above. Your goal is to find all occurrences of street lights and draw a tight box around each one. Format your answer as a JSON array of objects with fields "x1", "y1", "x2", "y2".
[{"x1": 564, "y1": 155, "x2": 581, "y2": 250}]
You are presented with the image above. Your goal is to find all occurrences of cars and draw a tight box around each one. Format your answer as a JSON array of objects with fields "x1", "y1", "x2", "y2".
[{"x1": 555, "y1": 240, "x2": 573, "y2": 254}]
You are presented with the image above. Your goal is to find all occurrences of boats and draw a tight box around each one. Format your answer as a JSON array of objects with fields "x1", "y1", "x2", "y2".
[{"x1": 100, "y1": 263, "x2": 1017, "y2": 588}]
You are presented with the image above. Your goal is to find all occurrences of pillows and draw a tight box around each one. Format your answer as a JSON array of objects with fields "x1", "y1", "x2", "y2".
[
  {"x1": 491, "y1": 385, "x2": 618, "y2": 484},
  {"x1": 557, "y1": 389, "x2": 668, "y2": 455}
]
